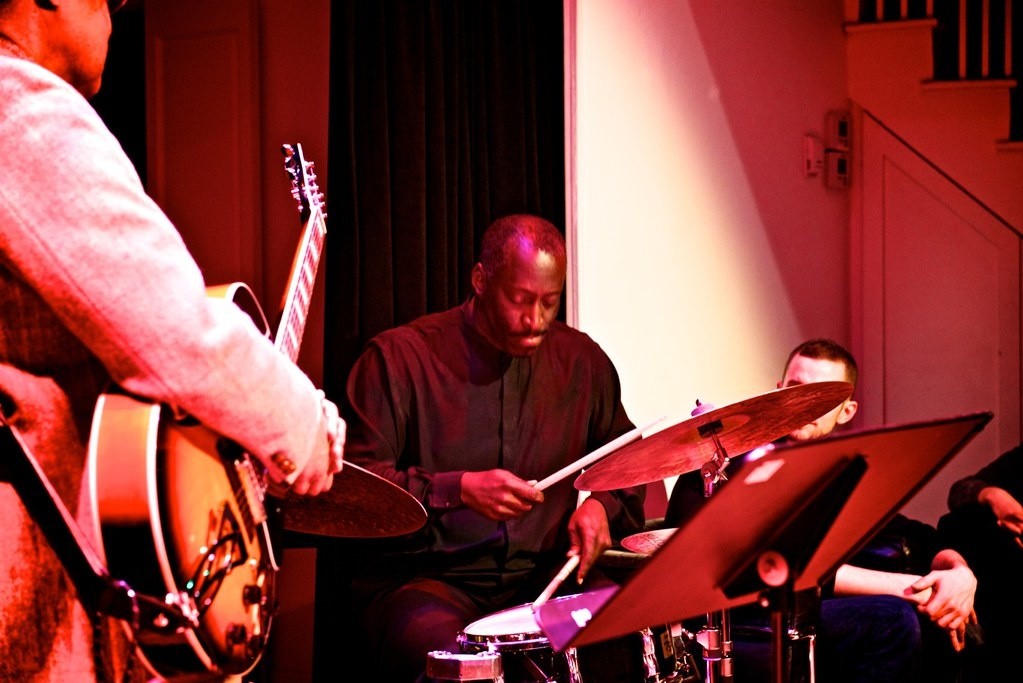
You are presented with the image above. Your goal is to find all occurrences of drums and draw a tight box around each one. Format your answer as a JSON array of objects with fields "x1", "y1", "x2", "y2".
[{"x1": 460, "y1": 584, "x2": 701, "y2": 682}]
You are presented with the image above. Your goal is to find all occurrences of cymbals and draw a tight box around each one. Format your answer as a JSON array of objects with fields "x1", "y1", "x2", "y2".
[
  {"x1": 621, "y1": 525, "x2": 677, "y2": 560},
  {"x1": 567, "y1": 381, "x2": 861, "y2": 494},
  {"x1": 268, "y1": 460, "x2": 428, "y2": 537}
]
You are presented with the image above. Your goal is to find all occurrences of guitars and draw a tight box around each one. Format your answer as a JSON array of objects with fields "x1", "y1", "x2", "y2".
[{"x1": 77, "y1": 130, "x2": 330, "y2": 683}]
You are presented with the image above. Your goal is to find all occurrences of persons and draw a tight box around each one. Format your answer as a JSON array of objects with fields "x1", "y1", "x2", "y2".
[
  {"x1": 662, "y1": 337, "x2": 984, "y2": 683},
  {"x1": 0, "y1": 0, "x2": 348, "y2": 683},
  {"x1": 343, "y1": 214, "x2": 648, "y2": 683},
  {"x1": 936, "y1": 440, "x2": 1023, "y2": 597}
]
